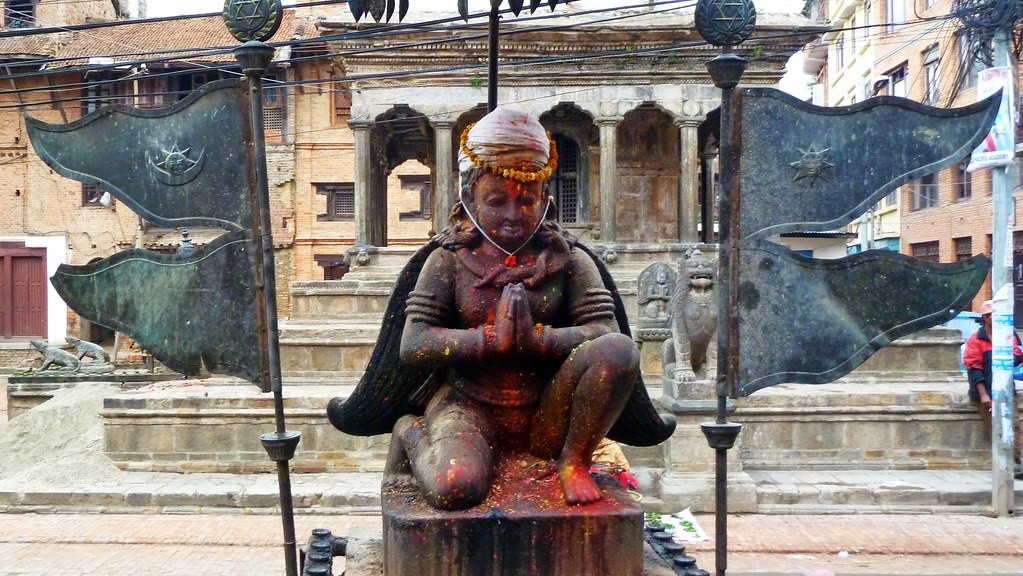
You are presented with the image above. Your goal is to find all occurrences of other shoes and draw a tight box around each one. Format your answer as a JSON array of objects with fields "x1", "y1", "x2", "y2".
[{"x1": 1014, "y1": 463, "x2": 1023, "y2": 475}]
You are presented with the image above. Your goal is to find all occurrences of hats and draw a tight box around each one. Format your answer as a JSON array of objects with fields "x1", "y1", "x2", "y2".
[{"x1": 981, "y1": 300, "x2": 993, "y2": 314}]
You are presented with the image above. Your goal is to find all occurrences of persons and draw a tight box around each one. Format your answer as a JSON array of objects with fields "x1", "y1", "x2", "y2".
[
  {"x1": 963, "y1": 299, "x2": 1023, "y2": 480},
  {"x1": 326, "y1": 107, "x2": 679, "y2": 511}
]
